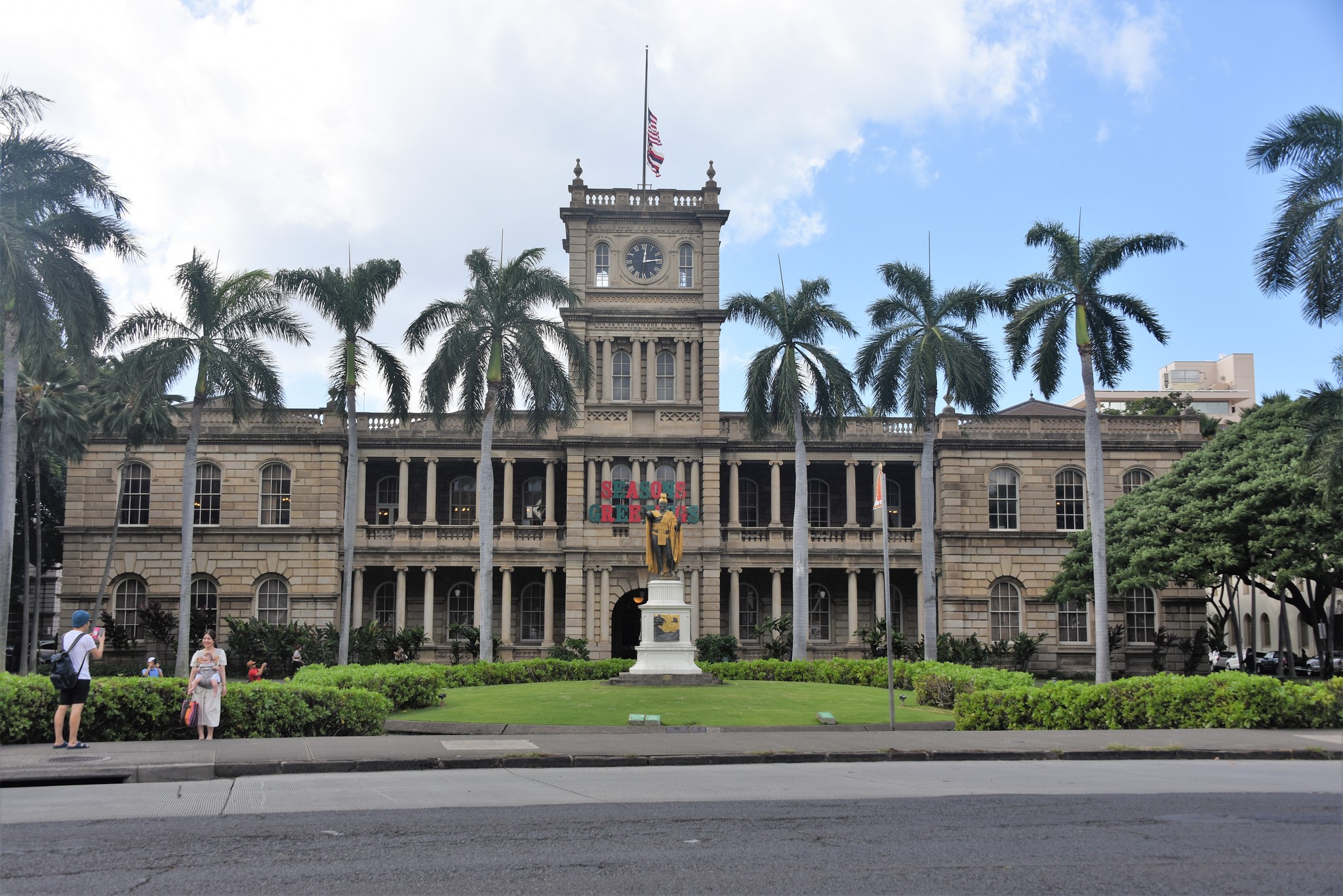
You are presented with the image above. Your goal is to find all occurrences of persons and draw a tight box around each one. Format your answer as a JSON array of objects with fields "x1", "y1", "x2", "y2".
[
  {"x1": 1242, "y1": 648, "x2": 1254, "y2": 674},
  {"x1": 247, "y1": 660, "x2": 266, "y2": 681},
  {"x1": 641, "y1": 493, "x2": 682, "y2": 577},
  {"x1": 1324, "y1": 650, "x2": 1333, "y2": 679},
  {"x1": 287, "y1": 644, "x2": 305, "y2": 678},
  {"x1": 189, "y1": 653, "x2": 220, "y2": 697},
  {"x1": 141, "y1": 657, "x2": 163, "y2": 677},
  {"x1": 187, "y1": 629, "x2": 227, "y2": 740},
  {"x1": 393, "y1": 642, "x2": 405, "y2": 665},
  {"x1": 1301, "y1": 648, "x2": 1305, "y2": 659},
  {"x1": 53, "y1": 609, "x2": 105, "y2": 750}
]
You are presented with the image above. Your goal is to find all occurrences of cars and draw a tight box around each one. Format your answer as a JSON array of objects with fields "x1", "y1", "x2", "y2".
[{"x1": 1208, "y1": 650, "x2": 1343, "y2": 678}]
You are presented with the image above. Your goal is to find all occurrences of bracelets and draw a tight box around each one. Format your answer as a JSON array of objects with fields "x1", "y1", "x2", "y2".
[{"x1": 222, "y1": 684, "x2": 226, "y2": 686}]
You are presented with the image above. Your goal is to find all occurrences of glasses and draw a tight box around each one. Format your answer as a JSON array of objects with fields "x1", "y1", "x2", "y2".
[
  {"x1": 88, "y1": 619, "x2": 93, "y2": 623},
  {"x1": 251, "y1": 663, "x2": 256, "y2": 666}
]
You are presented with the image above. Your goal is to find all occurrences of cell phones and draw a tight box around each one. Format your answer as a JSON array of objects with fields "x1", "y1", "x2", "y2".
[
  {"x1": 98, "y1": 628, "x2": 105, "y2": 637},
  {"x1": 154, "y1": 659, "x2": 158, "y2": 664}
]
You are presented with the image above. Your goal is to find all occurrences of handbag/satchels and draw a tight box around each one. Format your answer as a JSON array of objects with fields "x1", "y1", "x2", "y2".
[
  {"x1": 179, "y1": 693, "x2": 199, "y2": 727},
  {"x1": 292, "y1": 655, "x2": 302, "y2": 663},
  {"x1": 394, "y1": 653, "x2": 408, "y2": 660}
]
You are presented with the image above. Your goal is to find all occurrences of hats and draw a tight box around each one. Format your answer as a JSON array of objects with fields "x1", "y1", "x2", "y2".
[
  {"x1": 148, "y1": 657, "x2": 155, "y2": 662},
  {"x1": 71, "y1": 609, "x2": 90, "y2": 628},
  {"x1": 246, "y1": 660, "x2": 256, "y2": 667}
]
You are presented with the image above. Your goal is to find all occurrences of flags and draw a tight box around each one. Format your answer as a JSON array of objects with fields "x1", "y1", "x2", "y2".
[
  {"x1": 648, "y1": 107, "x2": 662, "y2": 146},
  {"x1": 647, "y1": 137, "x2": 664, "y2": 177}
]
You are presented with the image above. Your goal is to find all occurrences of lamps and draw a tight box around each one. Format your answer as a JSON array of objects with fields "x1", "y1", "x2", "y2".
[
  {"x1": 633, "y1": 595, "x2": 644, "y2": 603},
  {"x1": 818, "y1": 584, "x2": 826, "y2": 599},
  {"x1": 454, "y1": 581, "x2": 461, "y2": 597}
]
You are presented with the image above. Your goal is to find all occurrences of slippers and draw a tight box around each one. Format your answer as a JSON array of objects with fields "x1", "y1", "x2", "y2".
[
  {"x1": 66, "y1": 740, "x2": 89, "y2": 749},
  {"x1": 53, "y1": 740, "x2": 68, "y2": 748}
]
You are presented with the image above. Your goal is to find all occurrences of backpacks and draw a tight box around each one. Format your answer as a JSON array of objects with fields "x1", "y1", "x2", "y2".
[{"x1": 49, "y1": 631, "x2": 90, "y2": 691}]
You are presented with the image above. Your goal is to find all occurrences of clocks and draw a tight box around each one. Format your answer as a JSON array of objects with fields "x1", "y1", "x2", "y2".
[{"x1": 619, "y1": 236, "x2": 668, "y2": 286}]
